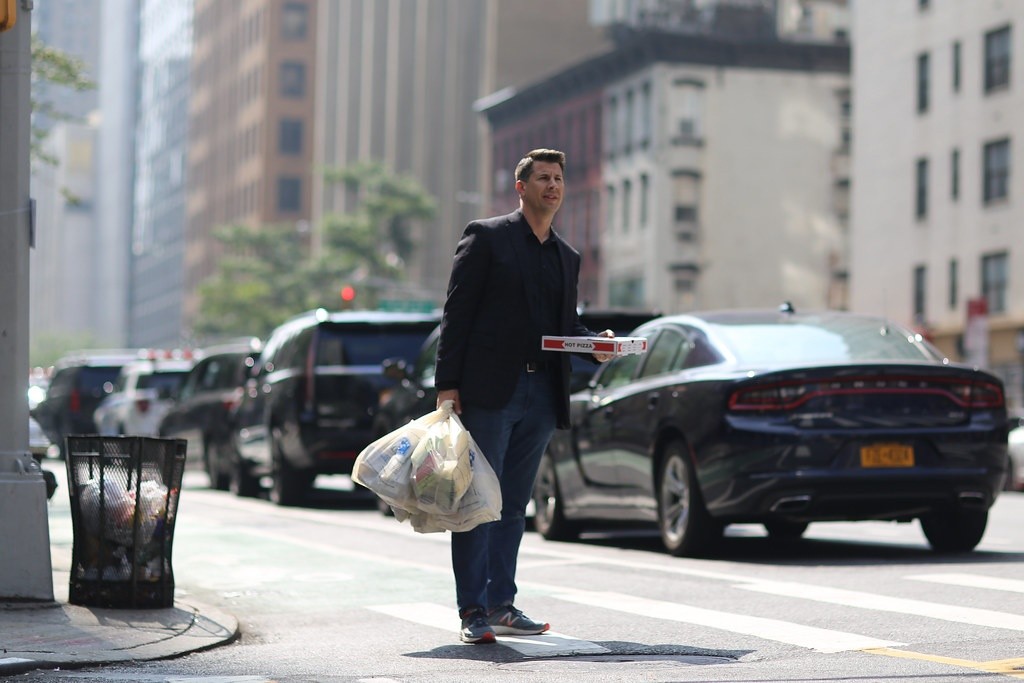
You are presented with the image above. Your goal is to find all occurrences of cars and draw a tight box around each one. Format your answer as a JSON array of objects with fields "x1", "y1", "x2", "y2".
[
  {"x1": 530, "y1": 301, "x2": 1010, "y2": 557},
  {"x1": 99, "y1": 363, "x2": 185, "y2": 458},
  {"x1": 362, "y1": 305, "x2": 665, "y2": 516},
  {"x1": 158, "y1": 344, "x2": 268, "y2": 488}
]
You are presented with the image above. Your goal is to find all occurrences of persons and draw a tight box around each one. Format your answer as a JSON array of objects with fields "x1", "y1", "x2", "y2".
[{"x1": 432, "y1": 147, "x2": 620, "y2": 645}]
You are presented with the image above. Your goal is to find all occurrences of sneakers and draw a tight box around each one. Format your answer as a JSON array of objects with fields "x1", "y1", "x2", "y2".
[
  {"x1": 458, "y1": 605, "x2": 496, "y2": 644},
  {"x1": 485, "y1": 602, "x2": 549, "y2": 634}
]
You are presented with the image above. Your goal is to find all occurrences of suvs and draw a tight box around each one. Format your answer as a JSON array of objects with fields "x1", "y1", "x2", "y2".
[
  {"x1": 28, "y1": 350, "x2": 157, "y2": 455},
  {"x1": 233, "y1": 305, "x2": 441, "y2": 504}
]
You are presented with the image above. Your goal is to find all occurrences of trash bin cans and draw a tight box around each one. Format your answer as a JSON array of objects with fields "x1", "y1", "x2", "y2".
[{"x1": 60, "y1": 434, "x2": 188, "y2": 611}]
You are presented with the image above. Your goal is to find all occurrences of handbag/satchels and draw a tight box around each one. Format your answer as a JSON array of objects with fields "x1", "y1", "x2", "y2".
[{"x1": 351, "y1": 400, "x2": 503, "y2": 534}]
